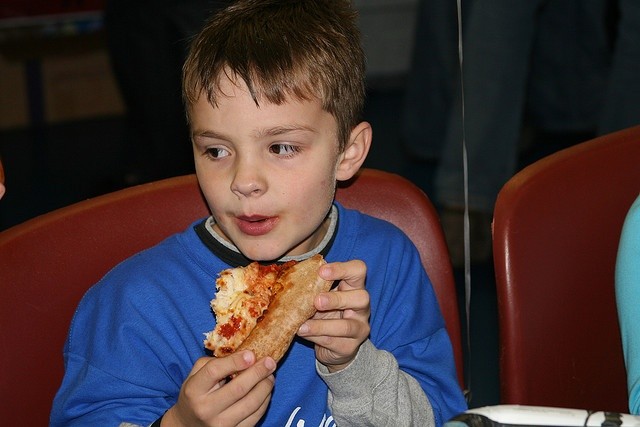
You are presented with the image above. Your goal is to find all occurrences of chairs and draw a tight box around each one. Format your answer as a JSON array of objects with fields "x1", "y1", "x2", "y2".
[
  {"x1": 491, "y1": 124, "x2": 640, "y2": 415},
  {"x1": 0, "y1": 169, "x2": 467, "y2": 426}
]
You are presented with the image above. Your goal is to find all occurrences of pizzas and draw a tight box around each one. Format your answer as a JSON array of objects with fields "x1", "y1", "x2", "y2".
[{"x1": 202, "y1": 253, "x2": 335, "y2": 380}]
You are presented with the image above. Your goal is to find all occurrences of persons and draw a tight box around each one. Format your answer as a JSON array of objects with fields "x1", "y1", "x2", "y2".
[
  {"x1": 615, "y1": 194, "x2": 638, "y2": 415},
  {"x1": 49, "y1": 0, "x2": 469, "y2": 427}
]
